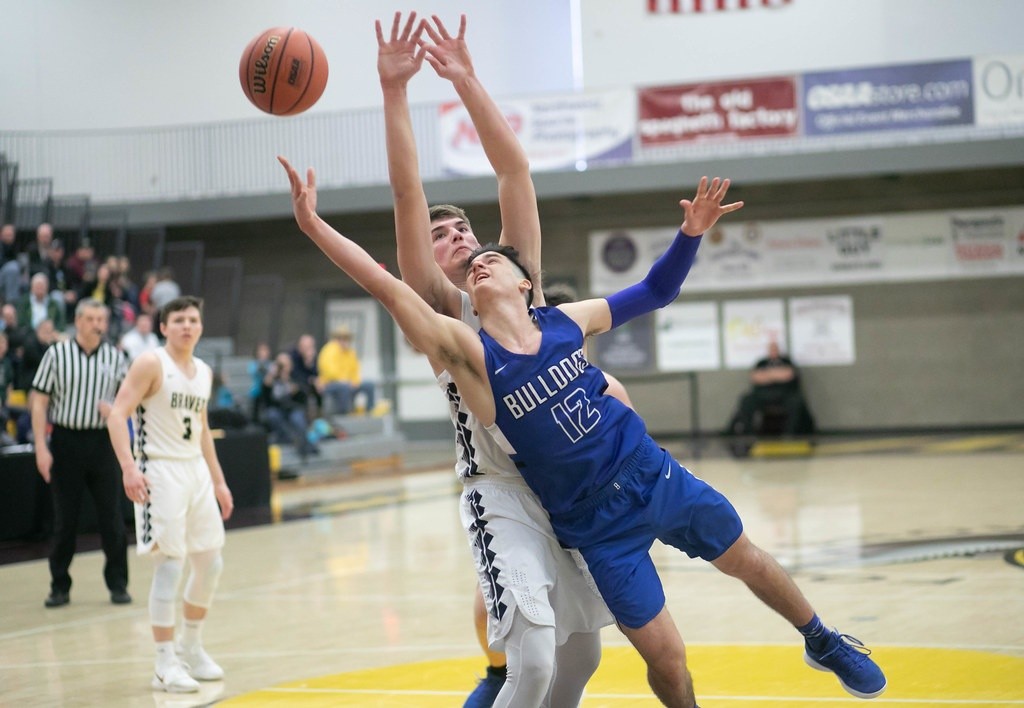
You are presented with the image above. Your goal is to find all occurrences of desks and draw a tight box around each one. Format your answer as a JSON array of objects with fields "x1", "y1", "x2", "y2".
[{"x1": 0, "y1": 430, "x2": 272, "y2": 544}]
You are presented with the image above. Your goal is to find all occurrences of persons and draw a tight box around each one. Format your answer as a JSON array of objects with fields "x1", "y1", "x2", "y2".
[
  {"x1": 108, "y1": 298, "x2": 236, "y2": 696},
  {"x1": 277, "y1": 9, "x2": 886, "y2": 708},
  {"x1": 743, "y1": 329, "x2": 813, "y2": 442},
  {"x1": 29, "y1": 298, "x2": 132, "y2": 609},
  {"x1": 7, "y1": 222, "x2": 379, "y2": 456}
]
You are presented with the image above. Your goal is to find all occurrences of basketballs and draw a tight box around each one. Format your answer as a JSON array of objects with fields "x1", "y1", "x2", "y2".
[{"x1": 236, "y1": 24, "x2": 330, "y2": 118}]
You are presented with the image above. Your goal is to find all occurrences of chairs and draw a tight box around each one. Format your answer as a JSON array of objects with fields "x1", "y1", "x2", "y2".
[{"x1": 190, "y1": 337, "x2": 403, "y2": 482}]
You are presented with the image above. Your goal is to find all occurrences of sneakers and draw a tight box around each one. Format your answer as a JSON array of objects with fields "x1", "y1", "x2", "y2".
[
  {"x1": 172, "y1": 641, "x2": 226, "y2": 681},
  {"x1": 150, "y1": 661, "x2": 198, "y2": 691},
  {"x1": 110, "y1": 587, "x2": 132, "y2": 605},
  {"x1": 44, "y1": 587, "x2": 71, "y2": 607},
  {"x1": 463, "y1": 673, "x2": 506, "y2": 708},
  {"x1": 803, "y1": 629, "x2": 889, "y2": 699}
]
